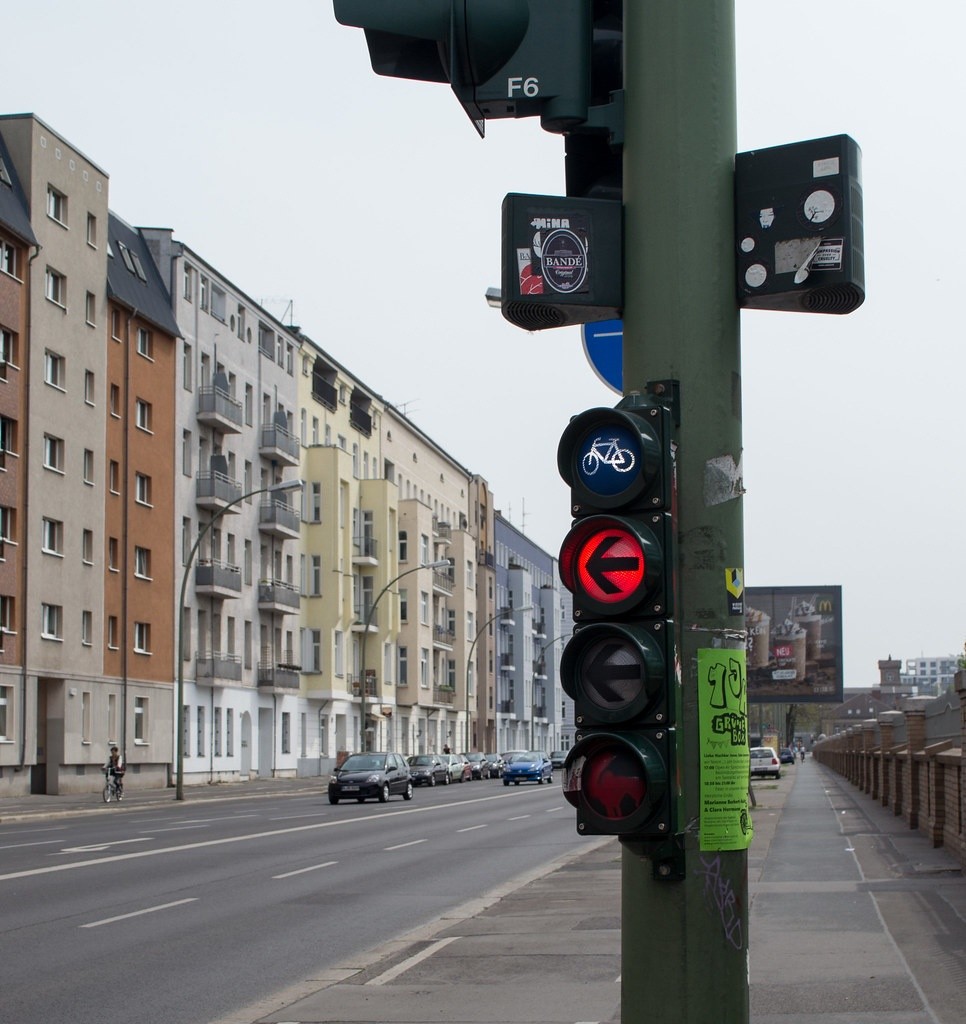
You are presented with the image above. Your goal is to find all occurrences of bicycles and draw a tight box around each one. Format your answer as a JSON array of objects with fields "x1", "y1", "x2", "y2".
[{"x1": 102, "y1": 767, "x2": 125, "y2": 804}]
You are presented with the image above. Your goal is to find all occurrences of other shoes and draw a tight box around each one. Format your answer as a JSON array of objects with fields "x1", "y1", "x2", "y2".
[{"x1": 120, "y1": 792, "x2": 123, "y2": 798}]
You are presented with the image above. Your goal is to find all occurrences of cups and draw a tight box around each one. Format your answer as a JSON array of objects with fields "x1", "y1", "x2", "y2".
[
  {"x1": 774, "y1": 629, "x2": 806, "y2": 681},
  {"x1": 799, "y1": 615, "x2": 822, "y2": 659},
  {"x1": 745, "y1": 617, "x2": 770, "y2": 669}
]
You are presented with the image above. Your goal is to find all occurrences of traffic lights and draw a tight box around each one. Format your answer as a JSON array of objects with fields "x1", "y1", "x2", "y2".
[
  {"x1": 558, "y1": 401, "x2": 677, "y2": 838},
  {"x1": 334, "y1": 1, "x2": 581, "y2": 137}
]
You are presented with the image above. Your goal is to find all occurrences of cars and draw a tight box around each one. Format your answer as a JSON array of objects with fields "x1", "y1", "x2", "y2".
[
  {"x1": 438, "y1": 754, "x2": 466, "y2": 785},
  {"x1": 503, "y1": 751, "x2": 554, "y2": 786},
  {"x1": 549, "y1": 751, "x2": 570, "y2": 770},
  {"x1": 460, "y1": 756, "x2": 473, "y2": 781},
  {"x1": 749, "y1": 748, "x2": 780, "y2": 779},
  {"x1": 460, "y1": 753, "x2": 491, "y2": 781},
  {"x1": 406, "y1": 754, "x2": 451, "y2": 787},
  {"x1": 328, "y1": 752, "x2": 414, "y2": 805},
  {"x1": 484, "y1": 753, "x2": 505, "y2": 779},
  {"x1": 775, "y1": 749, "x2": 795, "y2": 764}
]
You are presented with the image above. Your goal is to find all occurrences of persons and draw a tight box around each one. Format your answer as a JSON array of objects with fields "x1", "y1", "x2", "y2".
[
  {"x1": 800, "y1": 745, "x2": 806, "y2": 761},
  {"x1": 442, "y1": 744, "x2": 452, "y2": 755},
  {"x1": 103, "y1": 747, "x2": 125, "y2": 798}
]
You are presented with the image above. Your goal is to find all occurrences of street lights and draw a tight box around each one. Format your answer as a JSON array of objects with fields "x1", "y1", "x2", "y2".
[
  {"x1": 361, "y1": 560, "x2": 451, "y2": 755},
  {"x1": 532, "y1": 633, "x2": 573, "y2": 751},
  {"x1": 176, "y1": 479, "x2": 303, "y2": 801},
  {"x1": 465, "y1": 605, "x2": 535, "y2": 752}
]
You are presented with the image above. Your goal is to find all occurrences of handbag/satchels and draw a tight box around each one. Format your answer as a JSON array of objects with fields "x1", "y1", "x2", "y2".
[{"x1": 110, "y1": 768, "x2": 125, "y2": 778}]
